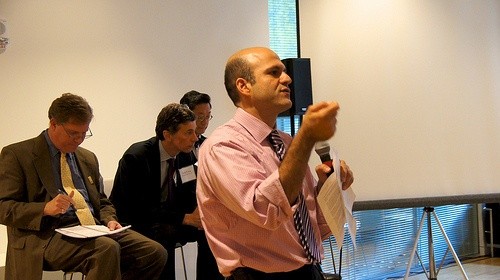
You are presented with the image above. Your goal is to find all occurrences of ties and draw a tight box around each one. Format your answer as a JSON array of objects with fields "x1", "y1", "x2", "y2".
[
  {"x1": 166, "y1": 158, "x2": 177, "y2": 225},
  {"x1": 61, "y1": 152, "x2": 96, "y2": 225},
  {"x1": 268, "y1": 129, "x2": 321, "y2": 264}
]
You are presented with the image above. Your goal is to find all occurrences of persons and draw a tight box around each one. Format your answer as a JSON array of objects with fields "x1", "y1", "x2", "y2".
[
  {"x1": 196, "y1": 46, "x2": 355, "y2": 280},
  {"x1": 179, "y1": 90, "x2": 213, "y2": 170},
  {"x1": 109, "y1": 104, "x2": 224, "y2": 280},
  {"x1": 0, "y1": 93, "x2": 167, "y2": 280}
]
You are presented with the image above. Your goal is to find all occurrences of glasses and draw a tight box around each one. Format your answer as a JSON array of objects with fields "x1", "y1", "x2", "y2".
[
  {"x1": 196, "y1": 114, "x2": 213, "y2": 121},
  {"x1": 59, "y1": 120, "x2": 92, "y2": 140}
]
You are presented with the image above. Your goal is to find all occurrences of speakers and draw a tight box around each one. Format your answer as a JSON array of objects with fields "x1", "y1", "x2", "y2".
[{"x1": 278, "y1": 57, "x2": 313, "y2": 117}]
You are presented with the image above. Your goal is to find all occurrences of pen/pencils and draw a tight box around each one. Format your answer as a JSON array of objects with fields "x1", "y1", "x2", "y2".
[{"x1": 58, "y1": 188, "x2": 76, "y2": 207}]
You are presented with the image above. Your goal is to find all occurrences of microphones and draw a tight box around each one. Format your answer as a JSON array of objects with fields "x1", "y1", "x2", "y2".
[{"x1": 315, "y1": 141, "x2": 334, "y2": 178}]
